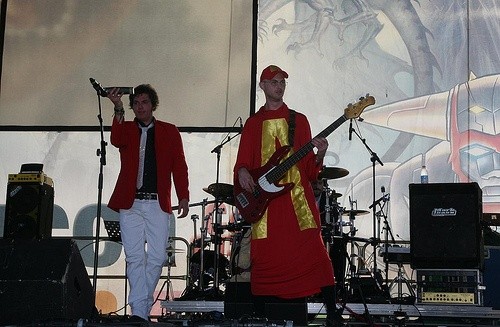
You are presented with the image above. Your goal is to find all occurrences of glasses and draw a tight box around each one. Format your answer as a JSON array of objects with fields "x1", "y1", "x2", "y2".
[{"x1": 271, "y1": 81, "x2": 288, "y2": 86}]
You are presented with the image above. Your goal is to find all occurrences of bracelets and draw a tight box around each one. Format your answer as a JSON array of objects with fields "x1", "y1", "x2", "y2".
[{"x1": 114, "y1": 107, "x2": 125, "y2": 114}]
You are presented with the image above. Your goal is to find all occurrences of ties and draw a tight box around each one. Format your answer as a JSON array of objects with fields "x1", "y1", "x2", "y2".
[{"x1": 136, "y1": 121, "x2": 154, "y2": 190}]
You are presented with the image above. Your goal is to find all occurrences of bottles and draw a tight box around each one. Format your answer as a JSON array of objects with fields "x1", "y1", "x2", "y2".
[{"x1": 420, "y1": 165, "x2": 428, "y2": 184}]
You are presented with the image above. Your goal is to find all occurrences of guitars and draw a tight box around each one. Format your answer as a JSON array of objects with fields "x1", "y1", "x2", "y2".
[{"x1": 233, "y1": 93, "x2": 376, "y2": 223}]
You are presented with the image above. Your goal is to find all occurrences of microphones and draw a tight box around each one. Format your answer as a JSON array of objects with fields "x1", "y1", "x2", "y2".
[
  {"x1": 369, "y1": 194, "x2": 388, "y2": 209},
  {"x1": 89, "y1": 77, "x2": 108, "y2": 97},
  {"x1": 349, "y1": 118, "x2": 353, "y2": 140}
]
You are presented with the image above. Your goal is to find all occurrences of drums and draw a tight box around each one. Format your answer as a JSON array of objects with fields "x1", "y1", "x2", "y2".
[{"x1": 315, "y1": 191, "x2": 340, "y2": 234}]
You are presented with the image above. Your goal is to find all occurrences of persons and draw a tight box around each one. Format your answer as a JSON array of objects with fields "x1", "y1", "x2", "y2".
[
  {"x1": 234, "y1": 65, "x2": 343, "y2": 327},
  {"x1": 107, "y1": 84, "x2": 190, "y2": 320}
]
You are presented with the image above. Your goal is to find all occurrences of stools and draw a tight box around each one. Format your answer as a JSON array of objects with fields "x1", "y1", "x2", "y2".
[{"x1": 382, "y1": 252, "x2": 414, "y2": 296}]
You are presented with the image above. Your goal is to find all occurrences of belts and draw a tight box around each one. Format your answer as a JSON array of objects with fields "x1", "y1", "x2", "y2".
[{"x1": 135, "y1": 194, "x2": 157, "y2": 200}]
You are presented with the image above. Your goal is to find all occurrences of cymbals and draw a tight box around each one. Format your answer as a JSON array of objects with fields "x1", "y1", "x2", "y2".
[
  {"x1": 209, "y1": 182, "x2": 236, "y2": 206},
  {"x1": 318, "y1": 168, "x2": 350, "y2": 179},
  {"x1": 342, "y1": 209, "x2": 371, "y2": 216}
]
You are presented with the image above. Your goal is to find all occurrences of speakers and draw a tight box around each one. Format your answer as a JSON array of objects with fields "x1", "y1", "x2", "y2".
[
  {"x1": 223, "y1": 282, "x2": 308, "y2": 327},
  {"x1": 409, "y1": 182, "x2": 485, "y2": 270},
  {"x1": 0, "y1": 237, "x2": 93, "y2": 327},
  {"x1": 4, "y1": 183, "x2": 55, "y2": 247}
]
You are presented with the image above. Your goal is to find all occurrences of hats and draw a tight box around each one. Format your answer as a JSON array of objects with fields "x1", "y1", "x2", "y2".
[{"x1": 260, "y1": 65, "x2": 288, "y2": 80}]
